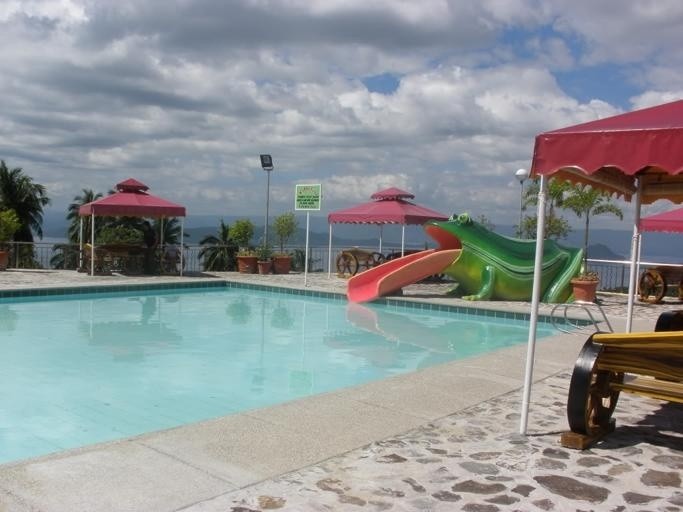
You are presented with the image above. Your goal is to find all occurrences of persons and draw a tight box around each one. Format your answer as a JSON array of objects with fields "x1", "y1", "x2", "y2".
[
  {"x1": 141, "y1": 219, "x2": 160, "y2": 274},
  {"x1": 133, "y1": 295, "x2": 157, "y2": 323}
]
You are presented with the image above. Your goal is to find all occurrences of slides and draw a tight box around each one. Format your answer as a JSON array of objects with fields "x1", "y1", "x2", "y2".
[{"x1": 347, "y1": 248, "x2": 461, "y2": 303}]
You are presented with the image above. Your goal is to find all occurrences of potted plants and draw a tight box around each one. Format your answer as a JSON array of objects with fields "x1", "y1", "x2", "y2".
[
  {"x1": 230, "y1": 214, "x2": 294, "y2": 274},
  {"x1": 0, "y1": 209, "x2": 22, "y2": 270},
  {"x1": 549, "y1": 176, "x2": 624, "y2": 303}
]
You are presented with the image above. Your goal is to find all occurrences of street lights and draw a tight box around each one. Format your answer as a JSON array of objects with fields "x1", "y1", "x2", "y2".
[
  {"x1": 260, "y1": 154, "x2": 274, "y2": 250},
  {"x1": 515, "y1": 168, "x2": 528, "y2": 240}
]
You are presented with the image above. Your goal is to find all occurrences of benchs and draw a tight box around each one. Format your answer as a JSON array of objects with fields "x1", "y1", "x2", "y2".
[
  {"x1": 76, "y1": 243, "x2": 185, "y2": 275},
  {"x1": 639, "y1": 266, "x2": 683, "y2": 305},
  {"x1": 561, "y1": 332, "x2": 683, "y2": 436},
  {"x1": 337, "y1": 248, "x2": 445, "y2": 284}
]
